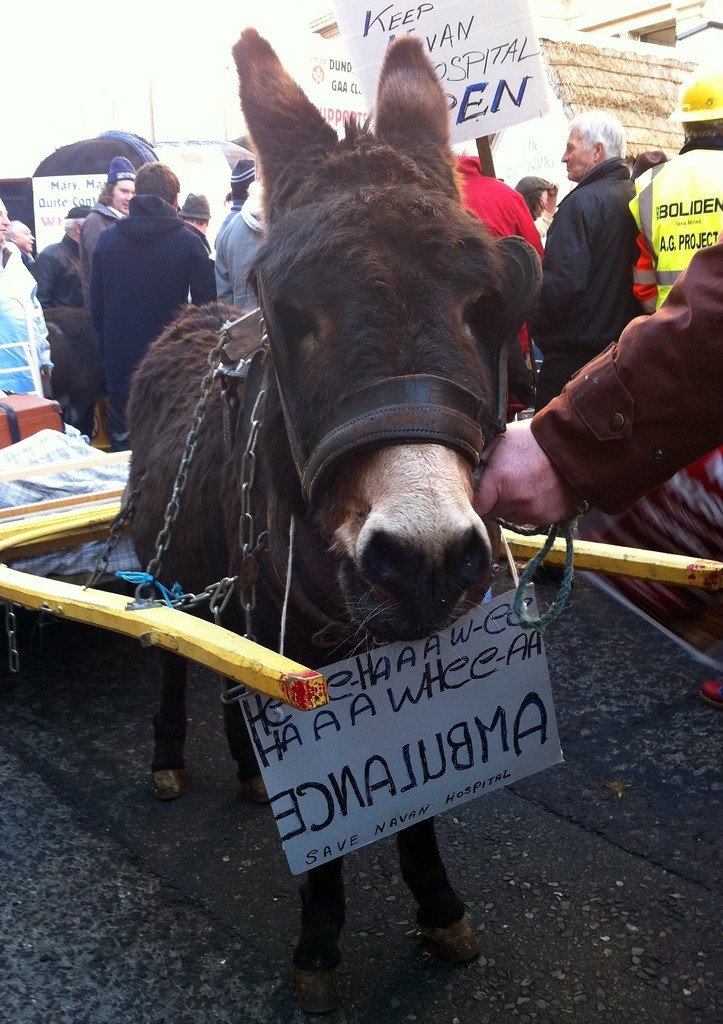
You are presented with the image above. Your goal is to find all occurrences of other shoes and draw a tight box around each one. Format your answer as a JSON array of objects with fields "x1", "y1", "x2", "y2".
[{"x1": 700, "y1": 679, "x2": 723, "y2": 710}]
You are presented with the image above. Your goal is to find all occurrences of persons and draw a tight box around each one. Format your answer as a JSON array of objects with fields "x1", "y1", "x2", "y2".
[
  {"x1": 455, "y1": 71, "x2": 723, "y2": 710},
  {"x1": 0, "y1": 157, "x2": 217, "y2": 453},
  {"x1": 214, "y1": 160, "x2": 266, "y2": 311}
]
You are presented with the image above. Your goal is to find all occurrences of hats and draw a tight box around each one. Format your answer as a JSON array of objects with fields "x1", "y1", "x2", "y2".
[
  {"x1": 515, "y1": 177, "x2": 552, "y2": 196},
  {"x1": 179, "y1": 194, "x2": 211, "y2": 219},
  {"x1": 630, "y1": 150, "x2": 667, "y2": 180},
  {"x1": 68, "y1": 205, "x2": 91, "y2": 218},
  {"x1": 231, "y1": 160, "x2": 256, "y2": 198},
  {"x1": 108, "y1": 156, "x2": 137, "y2": 183}
]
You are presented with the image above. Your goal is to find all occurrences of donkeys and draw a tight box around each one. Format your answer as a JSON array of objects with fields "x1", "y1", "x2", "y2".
[{"x1": 119, "y1": 29, "x2": 501, "y2": 1024}]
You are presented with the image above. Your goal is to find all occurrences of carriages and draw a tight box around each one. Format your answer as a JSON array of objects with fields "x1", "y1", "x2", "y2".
[{"x1": 0, "y1": 27, "x2": 723, "y2": 812}]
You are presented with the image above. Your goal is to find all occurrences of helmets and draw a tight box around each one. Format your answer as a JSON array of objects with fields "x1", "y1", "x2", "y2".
[{"x1": 667, "y1": 75, "x2": 723, "y2": 122}]
